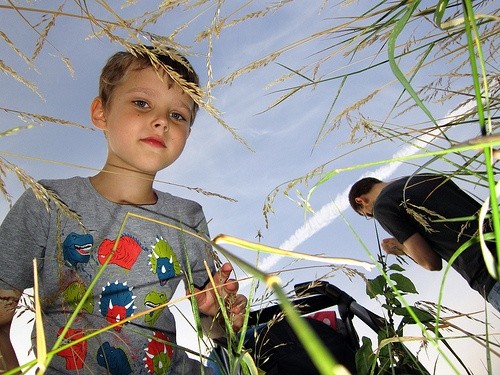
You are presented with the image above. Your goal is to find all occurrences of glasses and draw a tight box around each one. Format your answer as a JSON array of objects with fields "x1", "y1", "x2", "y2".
[{"x1": 357, "y1": 204, "x2": 373, "y2": 220}]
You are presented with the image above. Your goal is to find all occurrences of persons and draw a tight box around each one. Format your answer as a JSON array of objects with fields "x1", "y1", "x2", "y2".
[
  {"x1": 0, "y1": 44, "x2": 247, "y2": 375},
  {"x1": 349, "y1": 173, "x2": 500, "y2": 320}
]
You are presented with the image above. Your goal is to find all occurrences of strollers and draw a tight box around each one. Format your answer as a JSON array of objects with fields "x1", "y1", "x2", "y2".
[{"x1": 203, "y1": 279, "x2": 433, "y2": 375}]
